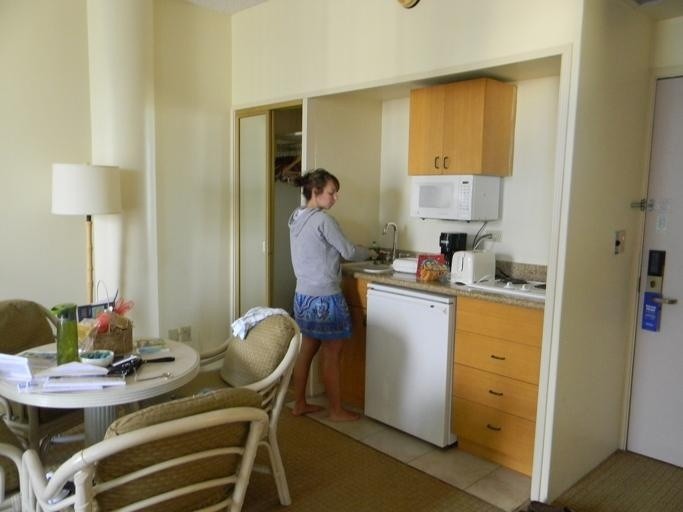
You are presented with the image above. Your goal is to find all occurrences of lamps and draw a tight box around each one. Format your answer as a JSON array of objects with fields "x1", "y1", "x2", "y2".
[{"x1": 52, "y1": 163, "x2": 122, "y2": 305}]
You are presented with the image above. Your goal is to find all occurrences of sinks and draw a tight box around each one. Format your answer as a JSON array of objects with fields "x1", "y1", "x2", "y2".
[{"x1": 340, "y1": 260, "x2": 393, "y2": 273}]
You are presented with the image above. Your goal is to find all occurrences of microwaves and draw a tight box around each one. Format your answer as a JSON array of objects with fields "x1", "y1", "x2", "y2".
[{"x1": 409, "y1": 173, "x2": 501, "y2": 221}]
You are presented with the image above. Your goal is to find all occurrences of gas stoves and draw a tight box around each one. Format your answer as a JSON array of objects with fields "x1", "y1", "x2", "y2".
[{"x1": 466, "y1": 278, "x2": 546, "y2": 301}]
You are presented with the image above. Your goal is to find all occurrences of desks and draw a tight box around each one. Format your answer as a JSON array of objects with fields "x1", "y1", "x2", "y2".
[{"x1": 0, "y1": 336, "x2": 200, "y2": 469}]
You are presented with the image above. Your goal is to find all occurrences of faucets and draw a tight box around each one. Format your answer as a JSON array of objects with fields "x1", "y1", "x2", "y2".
[{"x1": 381, "y1": 222, "x2": 399, "y2": 260}]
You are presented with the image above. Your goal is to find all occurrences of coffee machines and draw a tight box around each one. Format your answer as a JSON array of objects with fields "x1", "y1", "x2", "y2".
[{"x1": 438, "y1": 232, "x2": 467, "y2": 270}]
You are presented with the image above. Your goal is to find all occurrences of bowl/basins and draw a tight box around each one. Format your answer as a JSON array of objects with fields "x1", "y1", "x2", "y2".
[
  {"x1": 391, "y1": 258, "x2": 420, "y2": 274},
  {"x1": 81, "y1": 349, "x2": 115, "y2": 366}
]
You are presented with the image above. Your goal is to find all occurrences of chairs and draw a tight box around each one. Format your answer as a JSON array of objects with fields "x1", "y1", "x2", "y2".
[
  {"x1": 171, "y1": 307, "x2": 303, "y2": 507},
  {"x1": 1, "y1": 299, "x2": 123, "y2": 512},
  {"x1": 20, "y1": 387, "x2": 269, "y2": 512}
]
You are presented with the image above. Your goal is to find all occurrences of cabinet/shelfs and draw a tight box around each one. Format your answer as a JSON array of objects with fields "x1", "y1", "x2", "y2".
[
  {"x1": 408, "y1": 77, "x2": 517, "y2": 176},
  {"x1": 319, "y1": 279, "x2": 371, "y2": 409},
  {"x1": 450, "y1": 295, "x2": 544, "y2": 479}
]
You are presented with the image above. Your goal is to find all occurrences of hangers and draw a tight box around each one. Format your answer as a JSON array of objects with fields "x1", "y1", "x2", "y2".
[{"x1": 273, "y1": 154, "x2": 302, "y2": 185}]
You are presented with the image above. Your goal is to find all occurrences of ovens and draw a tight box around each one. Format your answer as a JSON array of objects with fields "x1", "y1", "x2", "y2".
[{"x1": 362, "y1": 283, "x2": 459, "y2": 447}]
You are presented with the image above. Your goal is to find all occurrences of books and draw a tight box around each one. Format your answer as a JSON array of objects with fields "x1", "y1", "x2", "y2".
[
  {"x1": 134, "y1": 335, "x2": 175, "y2": 361},
  {"x1": 15, "y1": 364, "x2": 127, "y2": 394}
]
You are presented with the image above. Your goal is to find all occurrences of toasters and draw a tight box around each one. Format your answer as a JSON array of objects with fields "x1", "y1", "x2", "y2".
[{"x1": 449, "y1": 248, "x2": 496, "y2": 285}]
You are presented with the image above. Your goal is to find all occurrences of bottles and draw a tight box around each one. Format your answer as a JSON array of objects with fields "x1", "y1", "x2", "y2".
[{"x1": 49, "y1": 302, "x2": 81, "y2": 366}]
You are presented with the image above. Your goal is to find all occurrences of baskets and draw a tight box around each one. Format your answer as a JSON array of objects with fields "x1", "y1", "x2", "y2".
[{"x1": 77, "y1": 312, "x2": 133, "y2": 357}]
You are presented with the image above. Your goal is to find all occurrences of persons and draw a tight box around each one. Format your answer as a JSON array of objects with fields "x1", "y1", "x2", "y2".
[{"x1": 287, "y1": 168, "x2": 380, "y2": 422}]
